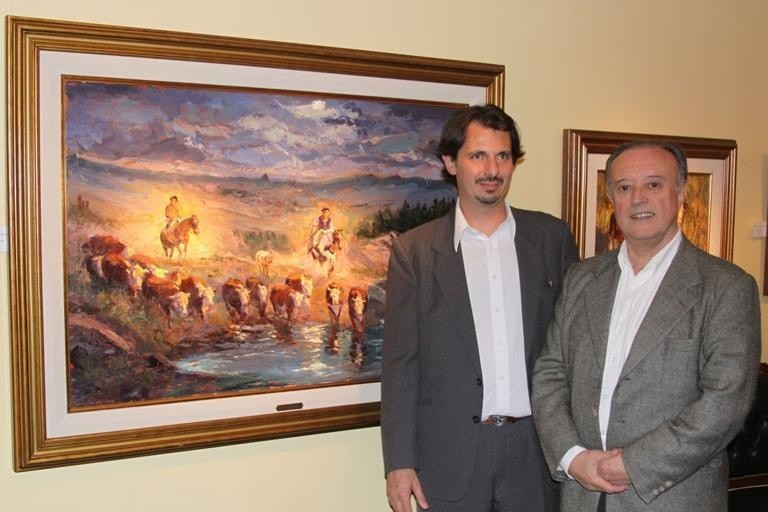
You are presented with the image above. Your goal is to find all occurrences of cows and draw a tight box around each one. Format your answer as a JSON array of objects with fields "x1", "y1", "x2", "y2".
[
  {"x1": 349, "y1": 288, "x2": 368, "y2": 333},
  {"x1": 222, "y1": 273, "x2": 313, "y2": 328},
  {"x1": 79, "y1": 234, "x2": 215, "y2": 329},
  {"x1": 326, "y1": 283, "x2": 345, "y2": 322}
]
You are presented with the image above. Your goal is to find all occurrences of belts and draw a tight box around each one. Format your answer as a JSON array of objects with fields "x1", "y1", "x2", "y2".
[{"x1": 481, "y1": 415, "x2": 531, "y2": 428}]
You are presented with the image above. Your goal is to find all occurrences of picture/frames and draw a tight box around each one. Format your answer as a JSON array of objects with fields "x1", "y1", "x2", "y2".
[
  {"x1": 4, "y1": 15, "x2": 506, "y2": 474},
  {"x1": 562, "y1": 128, "x2": 737, "y2": 263}
]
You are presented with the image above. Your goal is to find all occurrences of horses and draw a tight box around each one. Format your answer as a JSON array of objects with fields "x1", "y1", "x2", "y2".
[
  {"x1": 308, "y1": 227, "x2": 343, "y2": 280},
  {"x1": 159, "y1": 215, "x2": 200, "y2": 258}
]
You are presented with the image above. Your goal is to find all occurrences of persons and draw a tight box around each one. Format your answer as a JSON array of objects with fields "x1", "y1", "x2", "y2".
[
  {"x1": 530, "y1": 140, "x2": 762, "y2": 511},
  {"x1": 316, "y1": 206, "x2": 332, "y2": 229},
  {"x1": 379, "y1": 104, "x2": 579, "y2": 511},
  {"x1": 163, "y1": 196, "x2": 181, "y2": 228}
]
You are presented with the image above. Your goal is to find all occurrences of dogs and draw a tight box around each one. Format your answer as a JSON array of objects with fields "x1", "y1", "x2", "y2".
[{"x1": 255, "y1": 250, "x2": 272, "y2": 278}]
